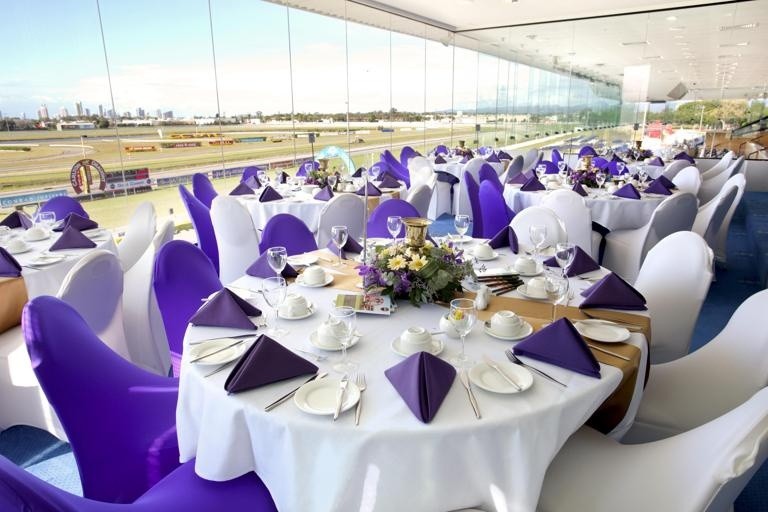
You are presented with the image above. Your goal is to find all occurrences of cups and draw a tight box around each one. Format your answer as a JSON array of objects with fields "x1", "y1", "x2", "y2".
[
  {"x1": 519, "y1": 257, "x2": 536, "y2": 272},
  {"x1": 477, "y1": 243, "x2": 494, "y2": 258}
]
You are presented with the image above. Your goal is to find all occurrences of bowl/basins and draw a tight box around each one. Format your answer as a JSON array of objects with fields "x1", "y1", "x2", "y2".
[
  {"x1": 526, "y1": 276, "x2": 550, "y2": 296},
  {"x1": 317, "y1": 319, "x2": 347, "y2": 346},
  {"x1": 276, "y1": 293, "x2": 308, "y2": 316},
  {"x1": 400, "y1": 327, "x2": 433, "y2": 354},
  {"x1": 490, "y1": 310, "x2": 522, "y2": 337},
  {"x1": 440, "y1": 314, "x2": 460, "y2": 339},
  {"x1": 303, "y1": 267, "x2": 324, "y2": 283}
]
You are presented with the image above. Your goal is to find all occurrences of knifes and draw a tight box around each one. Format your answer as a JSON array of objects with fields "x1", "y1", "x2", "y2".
[
  {"x1": 459, "y1": 369, "x2": 481, "y2": 418},
  {"x1": 486, "y1": 358, "x2": 522, "y2": 392},
  {"x1": 263, "y1": 373, "x2": 327, "y2": 412},
  {"x1": 190, "y1": 333, "x2": 257, "y2": 343},
  {"x1": 332, "y1": 375, "x2": 348, "y2": 420}
]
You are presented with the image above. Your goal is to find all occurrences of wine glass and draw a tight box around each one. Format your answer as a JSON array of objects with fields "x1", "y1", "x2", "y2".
[
  {"x1": 541, "y1": 276, "x2": 570, "y2": 328},
  {"x1": 422, "y1": 141, "x2": 685, "y2": 192},
  {"x1": 531, "y1": 224, "x2": 546, "y2": 259},
  {"x1": 556, "y1": 243, "x2": 576, "y2": 277},
  {"x1": 260, "y1": 277, "x2": 288, "y2": 337},
  {"x1": 331, "y1": 226, "x2": 349, "y2": 264},
  {"x1": 266, "y1": 247, "x2": 289, "y2": 296},
  {"x1": 449, "y1": 299, "x2": 478, "y2": 361},
  {"x1": 252, "y1": 163, "x2": 388, "y2": 201},
  {"x1": 327, "y1": 306, "x2": 358, "y2": 373},
  {"x1": 387, "y1": 215, "x2": 401, "y2": 242},
  {"x1": 454, "y1": 214, "x2": 470, "y2": 244},
  {"x1": 39, "y1": 211, "x2": 56, "y2": 238}
]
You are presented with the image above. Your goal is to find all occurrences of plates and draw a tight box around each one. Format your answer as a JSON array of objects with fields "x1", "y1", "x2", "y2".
[
  {"x1": 469, "y1": 360, "x2": 533, "y2": 394},
  {"x1": 275, "y1": 302, "x2": 317, "y2": 320},
  {"x1": 191, "y1": 338, "x2": 246, "y2": 365},
  {"x1": 472, "y1": 250, "x2": 499, "y2": 261},
  {"x1": 310, "y1": 330, "x2": 360, "y2": 351},
  {"x1": 446, "y1": 235, "x2": 472, "y2": 243},
  {"x1": 391, "y1": 339, "x2": 445, "y2": 358},
  {"x1": 575, "y1": 318, "x2": 630, "y2": 343},
  {"x1": 295, "y1": 275, "x2": 334, "y2": 288},
  {"x1": 294, "y1": 380, "x2": 360, "y2": 416},
  {"x1": 287, "y1": 255, "x2": 317, "y2": 266},
  {"x1": 1, "y1": 223, "x2": 96, "y2": 267},
  {"x1": 482, "y1": 320, "x2": 534, "y2": 342},
  {"x1": 517, "y1": 285, "x2": 550, "y2": 300}
]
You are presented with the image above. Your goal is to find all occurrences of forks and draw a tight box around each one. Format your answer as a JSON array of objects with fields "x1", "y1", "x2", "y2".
[
  {"x1": 505, "y1": 350, "x2": 568, "y2": 388},
  {"x1": 354, "y1": 374, "x2": 368, "y2": 425}
]
